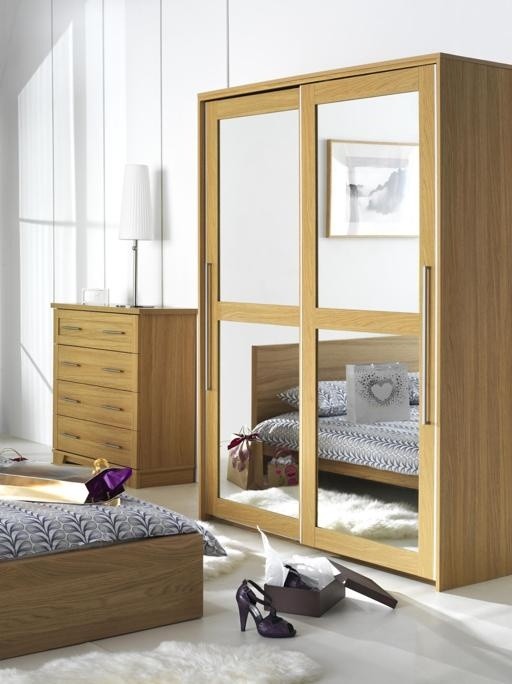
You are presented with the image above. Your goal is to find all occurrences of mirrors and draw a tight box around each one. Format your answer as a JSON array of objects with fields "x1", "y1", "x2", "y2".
[
  {"x1": 314, "y1": 327, "x2": 420, "y2": 556},
  {"x1": 313, "y1": 91, "x2": 420, "y2": 316},
  {"x1": 217, "y1": 320, "x2": 300, "y2": 524},
  {"x1": 216, "y1": 105, "x2": 303, "y2": 313}
]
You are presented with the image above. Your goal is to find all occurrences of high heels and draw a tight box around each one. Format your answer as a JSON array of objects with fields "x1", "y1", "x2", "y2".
[
  {"x1": 284, "y1": 565, "x2": 318, "y2": 592},
  {"x1": 236, "y1": 579, "x2": 296, "y2": 638}
]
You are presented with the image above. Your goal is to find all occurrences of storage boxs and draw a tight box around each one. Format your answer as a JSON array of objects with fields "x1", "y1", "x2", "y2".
[{"x1": 263, "y1": 560, "x2": 398, "y2": 619}]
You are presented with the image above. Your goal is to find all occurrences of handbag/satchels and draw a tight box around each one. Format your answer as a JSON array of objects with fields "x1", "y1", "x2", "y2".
[
  {"x1": 268, "y1": 462, "x2": 298, "y2": 487},
  {"x1": 227, "y1": 433, "x2": 264, "y2": 490}
]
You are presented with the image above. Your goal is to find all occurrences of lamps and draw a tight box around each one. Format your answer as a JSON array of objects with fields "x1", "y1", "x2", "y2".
[{"x1": 115, "y1": 164, "x2": 159, "y2": 310}]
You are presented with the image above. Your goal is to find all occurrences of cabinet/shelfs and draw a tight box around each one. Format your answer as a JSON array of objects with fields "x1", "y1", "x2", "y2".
[
  {"x1": 50, "y1": 302, "x2": 196, "y2": 490},
  {"x1": 198, "y1": 52, "x2": 511, "y2": 591}
]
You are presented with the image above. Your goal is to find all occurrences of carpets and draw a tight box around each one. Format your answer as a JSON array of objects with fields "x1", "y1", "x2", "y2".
[
  {"x1": 218, "y1": 480, "x2": 420, "y2": 541},
  {"x1": 0, "y1": 515, "x2": 324, "y2": 683}
]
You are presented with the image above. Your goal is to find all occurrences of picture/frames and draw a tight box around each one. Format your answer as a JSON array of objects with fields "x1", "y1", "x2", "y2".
[{"x1": 324, "y1": 138, "x2": 419, "y2": 242}]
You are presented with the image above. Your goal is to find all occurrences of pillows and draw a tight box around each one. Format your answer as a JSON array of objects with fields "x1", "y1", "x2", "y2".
[
  {"x1": 403, "y1": 372, "x2": 420, "y2": 405},
  {"x1": 276, "y1": 378, "x2": 346, "y2": 416}
]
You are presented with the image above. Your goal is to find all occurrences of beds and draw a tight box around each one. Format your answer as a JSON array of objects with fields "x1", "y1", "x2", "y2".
[
  {"x1": 0, "y1": 463, "x2": 224, "y2": 660},
  {"x1": 250, "y1": 335, "x2": 419, "y2": 490}
]
